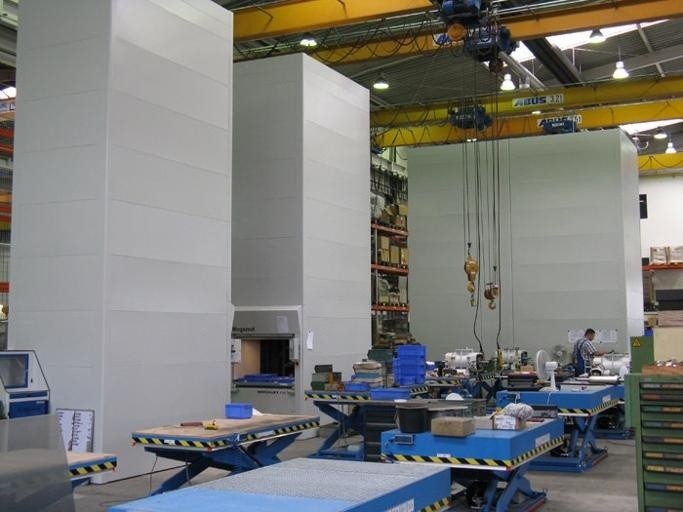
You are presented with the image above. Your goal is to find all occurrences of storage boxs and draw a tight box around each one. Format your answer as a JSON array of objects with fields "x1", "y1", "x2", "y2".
[
  {"x1": 649, "y1": 244, "x2": 683, "y2": 265},
  {"x1": 371, "y1": 191, "x2": 408, "y2": 306}
]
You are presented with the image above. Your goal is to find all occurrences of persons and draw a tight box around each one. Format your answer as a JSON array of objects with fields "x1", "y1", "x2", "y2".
[{"x1": 573, "y1": 328, "x2": 605, "y2": 377}]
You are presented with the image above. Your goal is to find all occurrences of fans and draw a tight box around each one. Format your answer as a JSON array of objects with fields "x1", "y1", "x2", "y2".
[{"x1": 534, "y1": 349, "x2": 560, "y2": 392}]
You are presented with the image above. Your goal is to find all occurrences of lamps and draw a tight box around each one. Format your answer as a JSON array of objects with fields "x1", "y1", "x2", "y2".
[
  {"x1": 299, "y1": 32, "x2": 389, "y2": 90},
  {"x1": 499, "y1": 27, "x2": 629, "y2": 91},
  {"x1": 654, "y1": 126, "x2": 677, "y2": 154}
]
